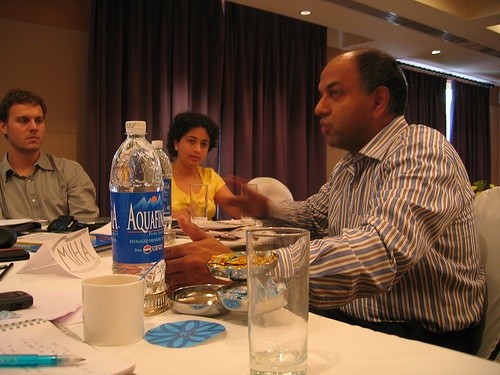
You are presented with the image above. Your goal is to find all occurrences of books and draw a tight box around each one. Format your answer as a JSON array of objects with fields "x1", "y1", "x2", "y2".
[
  {"x1": 16, "y1": 231, "x2": 112, "y2": 253},
  {"x1": 0, "y1": 317, "x2": 136, "y2": 375}
]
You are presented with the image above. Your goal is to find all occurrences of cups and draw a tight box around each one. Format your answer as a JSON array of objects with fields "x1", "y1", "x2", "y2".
[
  {"x1": 82, "y1": 274, "x2": 145, "y2": 347},
  {"x1": 190, "y1": 183, "x2": 208, "y2": 228},
  {"x1": 245, "y1": 227, "x2": 310, "y2": 375},
  {"x1": 240, "y1": 183, "x2": 258, "y2": 227}
]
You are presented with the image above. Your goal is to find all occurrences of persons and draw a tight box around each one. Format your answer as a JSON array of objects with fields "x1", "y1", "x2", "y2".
[
  {"x1": 0, "y1": 89, "x2": 98, "y2": 220},
  {"x1": 167, "y1": 112, "x2": 241, "y2": 219},
  {"x1": 165, "y1": 49, "x2": 487, "y2": 355}
]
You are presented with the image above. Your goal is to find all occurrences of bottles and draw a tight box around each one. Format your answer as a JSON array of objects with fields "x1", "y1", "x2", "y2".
[
  {"x1": 109, "y1": 120, "x2": 164, "y2": 296},
  {"x1": 153, "y1": 141, "x2": 173, "y2": 232}
]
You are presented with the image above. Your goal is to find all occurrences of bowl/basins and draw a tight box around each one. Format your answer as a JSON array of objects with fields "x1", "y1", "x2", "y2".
[
  {"x1": 168, "y1": 284, "x2": 229, "y2": 316},
  {"x1": 206, "y1": 252, "x2": 279, "y2": 282},
  {"x1": 218, "y1": 281, "x2": 290, "y2": 318}
]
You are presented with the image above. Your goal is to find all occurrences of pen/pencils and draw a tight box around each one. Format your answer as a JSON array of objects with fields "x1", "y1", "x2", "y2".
[
  {"x1": 18, "y1": 232, "x2": 30, "y2": 234},
  {"x1": 0, "y1": 264, "x2": 7, "y2": 269},
  {"x1": 0, "y1": 354, "x2": 86, "y2": 367},
  {"x1": 0, "y1": 263, "x2": 13, "y2": 281}
]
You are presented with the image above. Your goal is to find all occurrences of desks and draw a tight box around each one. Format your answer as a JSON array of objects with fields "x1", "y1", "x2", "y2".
[{"x1": 0, "y1": 218, "x2": 500, "y2": 375}]
